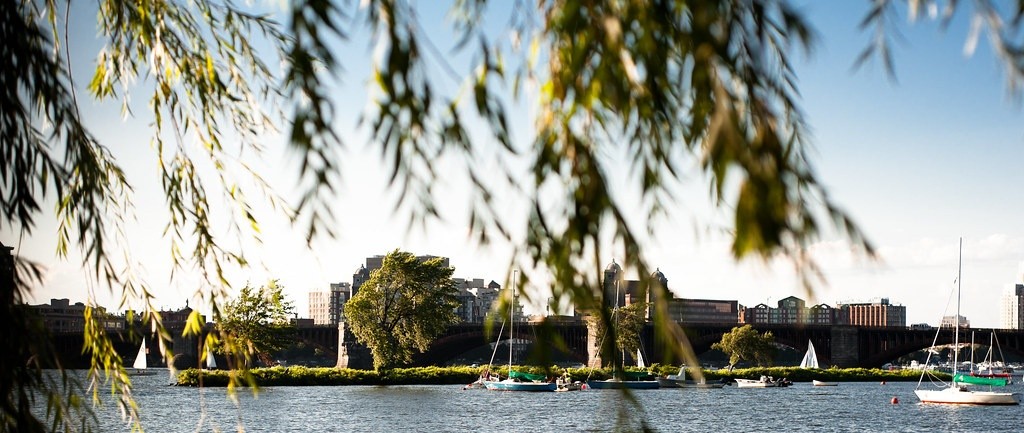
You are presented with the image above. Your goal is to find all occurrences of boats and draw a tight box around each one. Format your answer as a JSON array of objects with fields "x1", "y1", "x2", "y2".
[{"x1": 735, "y1": 375, "x2": 776, "y2": 389}]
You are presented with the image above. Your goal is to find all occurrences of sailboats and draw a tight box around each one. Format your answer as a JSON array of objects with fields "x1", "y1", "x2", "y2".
[
  {"x1": 584, "y1": 279, "x2": 661, "y2": 390},
  {"x1": 482, "y1": 268, "x2": 557, "y2": 392},
  {"x1": 913, "y1": 236, "x2": 1022, "y2": 406}
]
